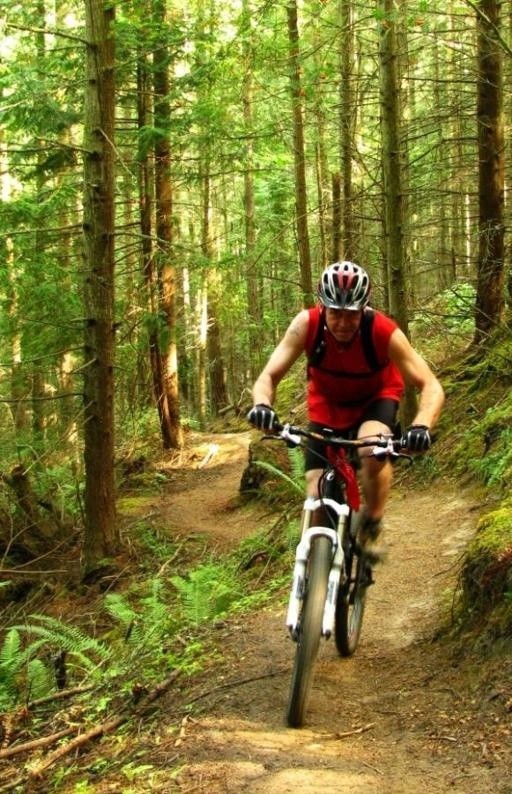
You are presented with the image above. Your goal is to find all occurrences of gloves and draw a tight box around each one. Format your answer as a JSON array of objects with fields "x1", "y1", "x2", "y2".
[
  {"x1": 248, "y1": 403, "x2": 279, "y2": 434},
  {"x1": 403, "y1": 425, "x2": 432, "y2": 451}
]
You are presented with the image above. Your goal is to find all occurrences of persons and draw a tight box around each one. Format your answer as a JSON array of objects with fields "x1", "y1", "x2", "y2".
[{"x1": 246, "y1": 259, "x2": 447, "y2": 564}]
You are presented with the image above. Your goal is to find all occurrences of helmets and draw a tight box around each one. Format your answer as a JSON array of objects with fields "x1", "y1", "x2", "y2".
[{"x1": 317, "y1": 261, "x2": 372, "y2": 311}]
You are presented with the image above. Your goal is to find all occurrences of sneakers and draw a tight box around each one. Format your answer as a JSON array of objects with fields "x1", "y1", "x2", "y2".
[{"x1": 350, "y1": 525, "x2": 381, "y2": 564}]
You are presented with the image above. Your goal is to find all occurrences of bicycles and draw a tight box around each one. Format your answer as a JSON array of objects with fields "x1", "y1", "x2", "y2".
[{"x1": 247, "y1": 411, "x2": 430, "y2": 726}]
